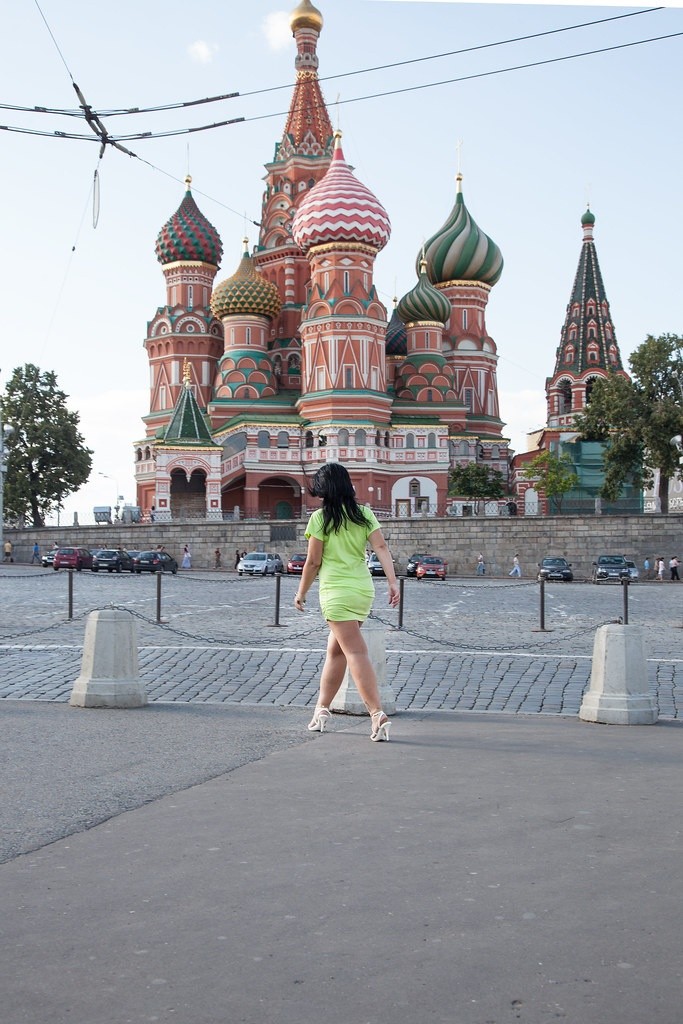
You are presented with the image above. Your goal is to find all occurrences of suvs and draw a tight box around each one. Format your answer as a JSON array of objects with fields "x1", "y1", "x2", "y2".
[{"x1": 591, "y1": 554, "x2": 633, "y2": 585}]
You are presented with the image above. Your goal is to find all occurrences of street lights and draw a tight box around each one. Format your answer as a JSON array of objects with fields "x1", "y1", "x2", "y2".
[{"x1": 98, "y1": 472, "x2": 119, "y2": 517}]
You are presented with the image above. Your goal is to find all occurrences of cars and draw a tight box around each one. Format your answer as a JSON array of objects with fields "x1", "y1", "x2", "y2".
[
  {"x1": 88, "y1": 549, "x2": 100, "y2": 557},
  {"x1": 406, "y1": 552, "x2": 432, "y2": 577},
  {"x1": 133, "y1": 550, "x2": 179, "y2": 574},
  {"x1": 536, "y1": 557, "x2": 574, "y2": 582},
  {"x1": 286, "y1": 553, "x2": 308, "y2": 575},
  {"x1": 414, "y1": 555, "x2": 448, "y2": 581},
  {"x1": 53, "y1": 546, "x2": 93, "y2": 571},
  {"x1": 366, "y1": 551, "x2": 396, "y2": 576},
  {"x1": 237, "y1": 551, "x2": 285, "y2": 577},
  {"x1": 125, "y1": 551, "x2": 142, "y2": 560},
  {"x1": 91, "y1": 548, "x2": 135, "y2": 573},
  {"x1": 626, "y1": 559, "x2": 641, "y2": 583},
  {"x1": 42, "y1": 548, "x2": 60, "y2": 567}
]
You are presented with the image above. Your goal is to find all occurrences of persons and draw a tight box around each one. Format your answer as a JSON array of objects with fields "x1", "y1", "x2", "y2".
[
  {"x1": 181, "y1": 548, "x2": 190, "y2": 569},
  {"x1": 214, "y1": 550, "x2": 220, "y2": 568},
  {"x1": 234, "y1": 549, "x2": 240, "y2": 569},
  {"x1": 476, "y1": 552, "x2": 483, "y2": 576},
  {"x1": 53, "y1": 542, "x2": 59, "y2": 550},
  {"x1": 508, "y1": 554, "x2": 521, "y2": 577},
  {"x1": 185, "y1": 545, "x2": 190, "y2": 553},
  {"x1": 241, "y1": 550, "x2": 247, "y2": 558},
  {"x1": 30, "y1": 543, "x2": 41, "y2": 564},
  {"x1": 641, "y1": 556, "x2": 681, "y2": 580},
  {"x1": 3, "y1": 540, "x2": 11, "y2": 562},
  {"x1": 294, "y1": 463, "x2": 400, "y2": 742}
]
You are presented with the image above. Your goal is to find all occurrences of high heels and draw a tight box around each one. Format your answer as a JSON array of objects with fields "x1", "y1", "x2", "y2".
[
  {"x1": 370, "y1": 711, "x2": 392, "y2": 741},
  {"x1": 308, "y1": 708, "x2": 332, "y2": 732}
]
